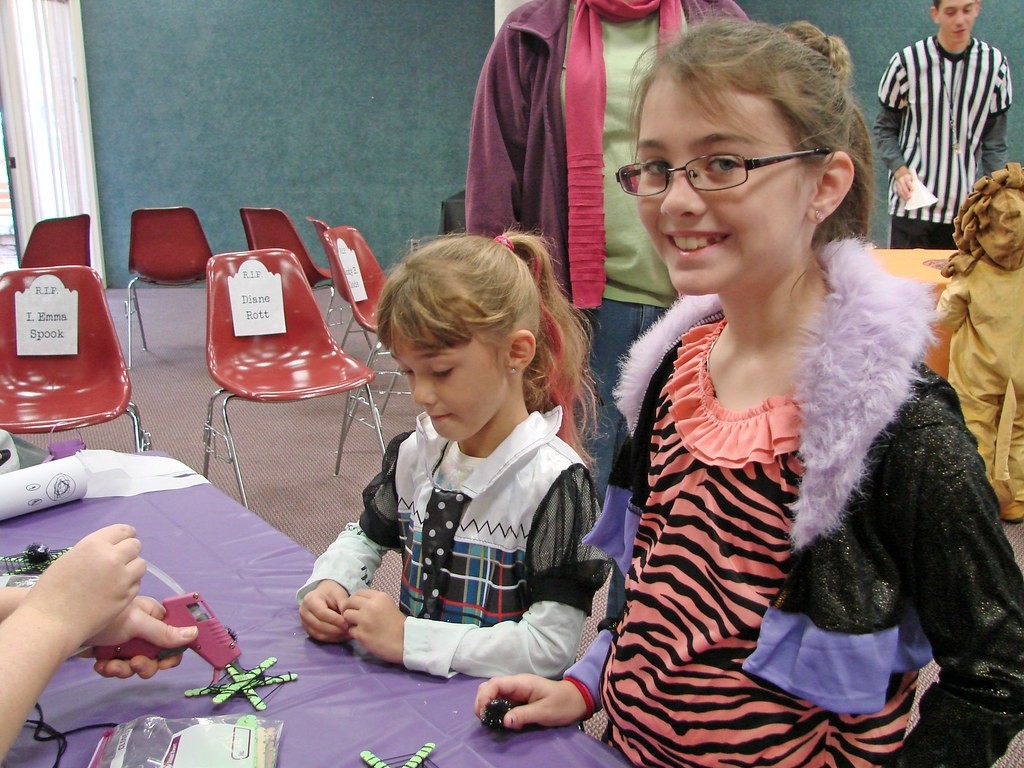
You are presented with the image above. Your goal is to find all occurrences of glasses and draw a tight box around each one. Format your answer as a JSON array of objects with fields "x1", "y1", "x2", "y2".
[{"x1": 618, "y1": 147, "x2": 830, "y2": 194}]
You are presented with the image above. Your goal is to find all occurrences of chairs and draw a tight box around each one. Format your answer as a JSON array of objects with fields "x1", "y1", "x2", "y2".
[
  {"x1": 201, "y1": 248, "x2": 388, "y2": 511},
  {"x1": 123, "y1": 206, "x2": 216, "y2": 370},
  {"x1": 0, "y1": 265, "x2": 153, "y2": 454},
  {"x1": 240, "y1": 206, "x2": 344, "y2": 327},
  {"x1": 307, "y1": 216, "x2": 350, "y2": 302},
  {"x1": 20, "y1": 214, "x2": 92, "y2": 266},
  {"x1": 322, "y1": 224, "x2": 389, "y2": 349}
]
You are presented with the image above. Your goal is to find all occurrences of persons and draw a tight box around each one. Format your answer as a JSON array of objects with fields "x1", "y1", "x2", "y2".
[
  {"x1": 474, "y1": 17, "x2": 1023, "y2": 768},
  {"x1": 297, "y1": 230, "x2": 611, "y2": 680},
  {"x1": 873, "y1": 1, "x2": 1011, "y2": 249},
  {"x1": 933, "y1": 163, "x2": 1024, "y2": 522},
  {"x1": 465, "y1": 1, "x2": 751, "y2": 503},
  {"x1": 0, "y1": 524, "x2": 198, "y2": 764}
]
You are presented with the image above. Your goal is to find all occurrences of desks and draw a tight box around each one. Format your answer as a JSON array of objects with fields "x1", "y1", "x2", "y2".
[
  {"x1": 873, "y1": 246, "x2": 962, "y2": 382},
  {"x1": 0, "y1": 447, "x2": 631, "y2": 767}
]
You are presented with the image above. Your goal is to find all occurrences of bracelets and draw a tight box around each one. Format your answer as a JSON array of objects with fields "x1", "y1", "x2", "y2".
[{"x1": 564, "y1": 677, "x2": 592, "y2": 719}]
